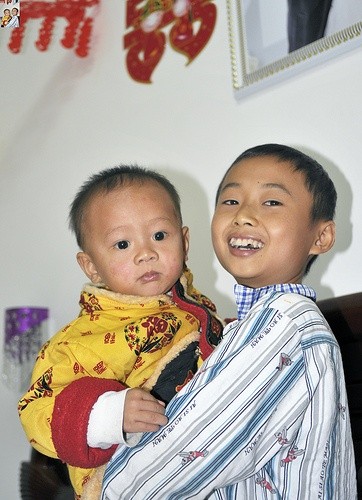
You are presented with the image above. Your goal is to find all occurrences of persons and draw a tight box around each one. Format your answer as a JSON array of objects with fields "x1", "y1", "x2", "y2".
[
  {"x1": 18, "y1": 163, "x2": 227, "y2": 500},
  {"x1": 102, "y1": 143, "x2": 357, "y2": 500}
]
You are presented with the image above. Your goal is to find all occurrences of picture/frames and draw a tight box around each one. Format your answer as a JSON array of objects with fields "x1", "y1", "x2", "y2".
[{"x1": 227, "y1": 0, "x2": 362, "y2": 101}]
